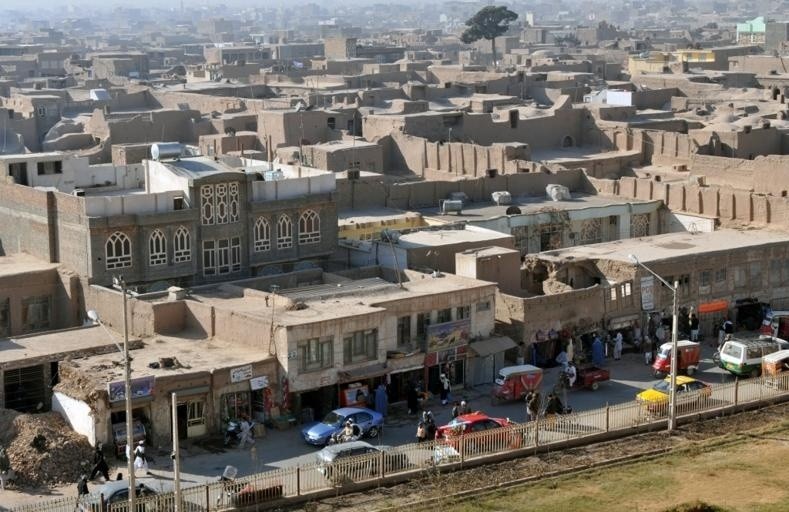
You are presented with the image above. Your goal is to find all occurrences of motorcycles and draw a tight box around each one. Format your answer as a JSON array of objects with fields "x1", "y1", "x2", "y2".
[
  {"x1": 223, "y1": 416, "x2": 256, "y2": 446},
  {"x1": 328, "y1": 432, "x2": 362, "y2": 445},
  {"x1": 217, "y1": 466, "x2": 249, "y2": 505}
]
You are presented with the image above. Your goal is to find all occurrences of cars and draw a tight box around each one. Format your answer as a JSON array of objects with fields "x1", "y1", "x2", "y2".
[
  {"x1": 110, "y1": 418, "x2": 146, "y2": 457},
  {"x1": 435, "y1": 414, "x2": 525, "y2": 453},
  {"x1": 634, "y1": 374, "x2": 711, "y2": 416},
  {"x1": 80, "y1": 480, "x2": 177, "y2": 512},
  {"x1": 301, "y1": 406, "x2": 384, "y2": 446}
]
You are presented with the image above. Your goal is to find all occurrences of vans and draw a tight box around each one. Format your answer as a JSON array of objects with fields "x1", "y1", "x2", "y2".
[
  {"x1": 716, "y1": 334, "x2": 789, "y2": 379},
  {"x1": 760, "y1": 311, "x2": 789, "y2": 339}
]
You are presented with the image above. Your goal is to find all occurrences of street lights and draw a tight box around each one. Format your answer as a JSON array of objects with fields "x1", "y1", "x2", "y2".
[
  {"x1": 86, "y1": 310, "x2": 137, "y2": 511},
  {"x1": 626, "y1": 254, "x2": 679, "y2": 434}
]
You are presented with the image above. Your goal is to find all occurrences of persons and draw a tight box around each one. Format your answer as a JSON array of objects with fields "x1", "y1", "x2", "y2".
[
  {"x1": 416, "y1": 404, "x2": 437, "y2": 442},
  {"x1": 407, "y1": 384, "x2": 423, "y2": 414},
  {"x1": 565, "y1": 362, "x2": 576, "y2": 387},
  {"x1": 0, "y1": 447, "x2": 11, "y2": 492},
  {"x1": 88, "y1": 447, "x2": 109, "y2": 482},
  {"x1": 613, "y1": 333, "x2": 622, "y2": 361},
  {"x1": 655, "y1": 326, "x2": 664, "y2": 343},
  {"x1": 591, "y1": 338, "x2": 604, "y2": 368},
  {"x1": 338, "y1": 418, "x2": 363, "y2": 442},
  {"x1": 644, "y1": 336, "x2": 652, "y2": 366},
  {"x1": 723, "y1": 319, "x2": 734, "y2": 341},
  {"x1": 525, "y1": 390, "x2": 563, "y2": 421},
  {"x1": 450, "y1": 401, "x2": 471, "y2": 420},
  {"x1": 690, "y1": 314, "x2": 699, "y2": 341},
  {"x1": 664, "y1": 325, "x2": 670, "y2": 342},
  {"x1": 134, "y1": 440, "x2": 153, "y2": 477},
  {"x1": 440, "y1": 373, "x2": 452, "y2": 406},
  {"x1": 75, "y1": 475, "x2": 89, "y2": 507},
  {"x1": 96, "y1": 440, "x2": 106, "y2": 459},
  {"x1": 221, "y1": 416, "x2": 256, "y2": 449}
]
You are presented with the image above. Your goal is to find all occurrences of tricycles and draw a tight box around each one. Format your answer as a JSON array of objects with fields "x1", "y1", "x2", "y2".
[
  {"x1": 652, "y1": 340, "x2": 700, "y2": 379},
  {"x1": 490, "y1": 364, "x2": 543, "y2": 406},
  {"x1": 551, "y1": 363, "x2": 610, "y2": 396}
]
